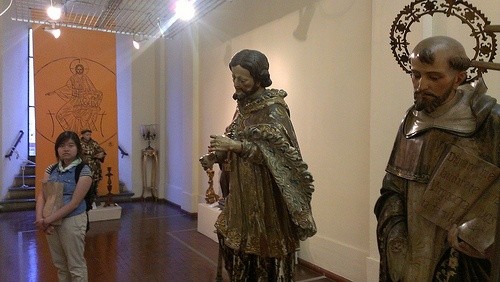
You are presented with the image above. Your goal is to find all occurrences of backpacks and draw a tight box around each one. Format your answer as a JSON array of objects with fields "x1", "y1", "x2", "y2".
[{"x1": 50, "y1": 162, "x2": 96, "y2": 212}]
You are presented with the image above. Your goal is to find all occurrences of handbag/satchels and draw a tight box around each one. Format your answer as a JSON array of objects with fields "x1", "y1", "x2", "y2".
[{"x1": 35, "y1": 179, "x2": 64, "y2": 226}]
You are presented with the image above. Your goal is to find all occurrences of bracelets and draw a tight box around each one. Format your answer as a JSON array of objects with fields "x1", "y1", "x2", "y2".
[{"x1": 41, "y1": 218, "x2": 48, "y2": 225}]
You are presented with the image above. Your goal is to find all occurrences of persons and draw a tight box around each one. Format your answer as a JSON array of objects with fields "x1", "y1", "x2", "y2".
[
  {"x1": 79, "y1": 130, "x2": 107, "y2": 206},
  {"x1": 373, "y1": 36, "x2": 500, "y2": 282},
  {"x1": 33, "y1": 131, "x2": 94, "y2": 282},
  {"x1": 199, "y1": 48, "x2": 318, "y2": 282}
]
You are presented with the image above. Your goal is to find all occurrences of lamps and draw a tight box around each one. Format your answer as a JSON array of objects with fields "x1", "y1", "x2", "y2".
[
  {"x1": 42, "y1": 27, "x2": 61, "y2": 39},
  {"x1": 133, "y1": 38, "x2": 149, "y2": 50}
]
